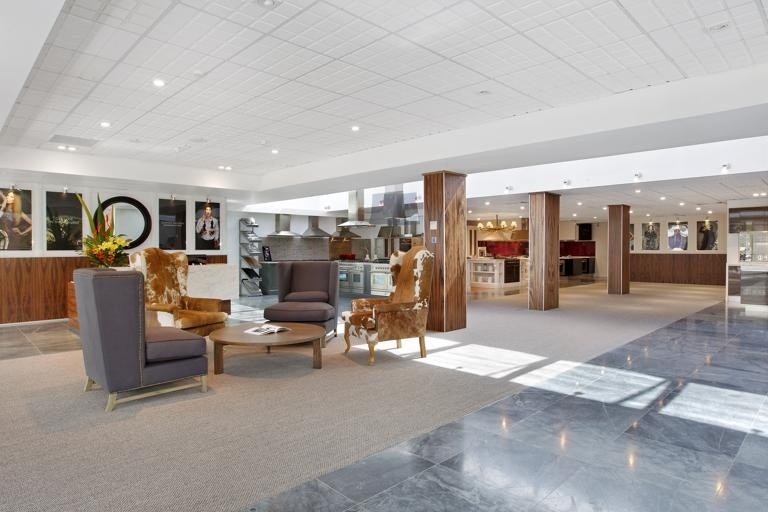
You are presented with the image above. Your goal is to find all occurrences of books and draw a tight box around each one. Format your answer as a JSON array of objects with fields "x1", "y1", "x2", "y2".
[
  {"x1": 240, "y1": 231, "x2": 263, "y2": 293},
  {"x1": 244, "y1": 324, "x2": 291, "y2": 336}
]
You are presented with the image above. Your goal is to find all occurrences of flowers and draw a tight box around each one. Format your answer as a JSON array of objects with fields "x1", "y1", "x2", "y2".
[{"x1": 73, "y1": 187, "x2": 133, "y2": 262}]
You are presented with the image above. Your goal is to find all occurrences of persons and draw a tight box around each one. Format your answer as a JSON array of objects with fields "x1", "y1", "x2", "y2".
[
  {"x1": 643, "y1": 225, "x2": 658, "y2": 249},
  {"x1": 196, "y1": 204, "x2": 220, "y2": 250},
  {"x1": 0, "y1": 189, "x2": 32, "y2": 249}
]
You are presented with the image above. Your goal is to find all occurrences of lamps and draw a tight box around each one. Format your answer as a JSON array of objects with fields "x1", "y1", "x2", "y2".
[{"x1": 476, "y1": 213, "x2": 519, "y2": 234}]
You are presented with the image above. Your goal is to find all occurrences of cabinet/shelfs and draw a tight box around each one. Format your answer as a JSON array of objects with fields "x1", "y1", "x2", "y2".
[{"x1": 238, "y1": 217, "x2": 263, "y2": 297}]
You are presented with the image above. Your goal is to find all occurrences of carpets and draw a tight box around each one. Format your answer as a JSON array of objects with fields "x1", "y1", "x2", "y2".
[{"x1": 0, "y1": 281, "x2": 723, "y2": 512}]
